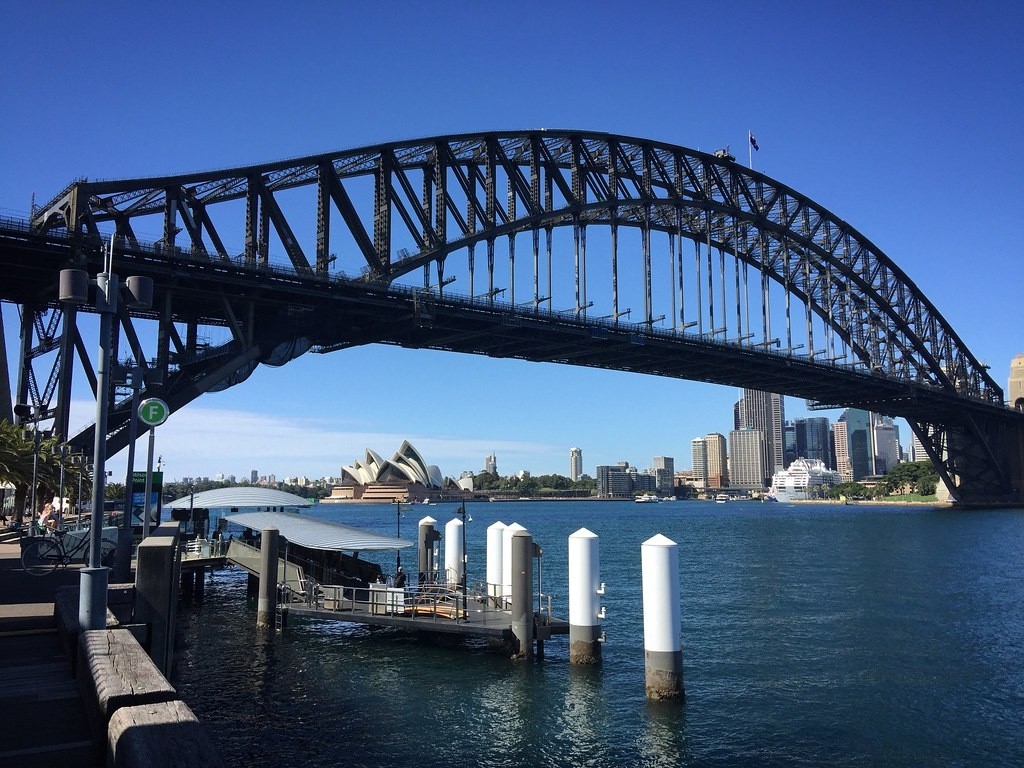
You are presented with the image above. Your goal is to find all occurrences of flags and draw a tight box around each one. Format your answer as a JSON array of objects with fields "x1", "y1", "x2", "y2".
[{"x1": 750, "y1": 131, "x2": 760, "y2": 151}]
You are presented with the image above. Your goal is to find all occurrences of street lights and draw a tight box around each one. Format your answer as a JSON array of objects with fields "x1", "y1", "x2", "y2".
[
  {"x1": 72, "y1": 456, "x2": 92, "y2": 530},
  {"x1": 51, "y1": 446, "x2": 75, "y2": 533},
  {"x1": 22, "y1": 430, "x2": 53, "y2": 536},
  {"x1": 77, "y1": 465, "x2": 93, "y2": 532},
  {"x1": 59, "y1": 270, "x2": 155, "y2": 629},
  {"x1": 397, "y1": 507, "x2": 413, "y2": 572},
  {"x1": 453, "y1": 508, "x2": 467, "y2": 622},
  {"x1": 111, "y1": 364, "x2": 164, "y2": 583}
]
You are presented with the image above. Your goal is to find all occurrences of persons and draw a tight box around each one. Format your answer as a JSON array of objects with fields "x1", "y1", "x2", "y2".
[
  {"x1": 38, "y1": 502, "x2": 59, "y2": 533},
  {"x1": 393, "y1": 567, "x2": 406, "y2": 588},
  {"x1": 416, "y1": 571, "x2": 426, "y2": 594},
  {"x1": 212, "y1": 525, "x2": 223, "y2": 539}
]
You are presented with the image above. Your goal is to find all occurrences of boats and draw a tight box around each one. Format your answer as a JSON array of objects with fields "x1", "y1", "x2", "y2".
[
  {"x1": 635, "y1": 495, "x2": 659, "y2": 503},
  {"x1": 391, "y1": 495, "x2": 415, "y2": 505},
  {"x1": 422, "y1": 499, "x2": 430, "y2": 505},
  {"x1": 698, "y1": 493, "x2": 711, "y2": 501},
  {"x1": 715, "y1": 495, "x2": 729, "y2": 504}
]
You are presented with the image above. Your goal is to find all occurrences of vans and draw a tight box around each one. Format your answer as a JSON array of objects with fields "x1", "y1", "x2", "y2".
[{"x1": 52, "y1": 497, "x2": 78, "y2": 514}]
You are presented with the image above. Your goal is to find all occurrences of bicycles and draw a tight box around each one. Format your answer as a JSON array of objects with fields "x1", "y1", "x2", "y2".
[{"x1": 21, "y1": 523, "x2": 119, "y2": 577}]
